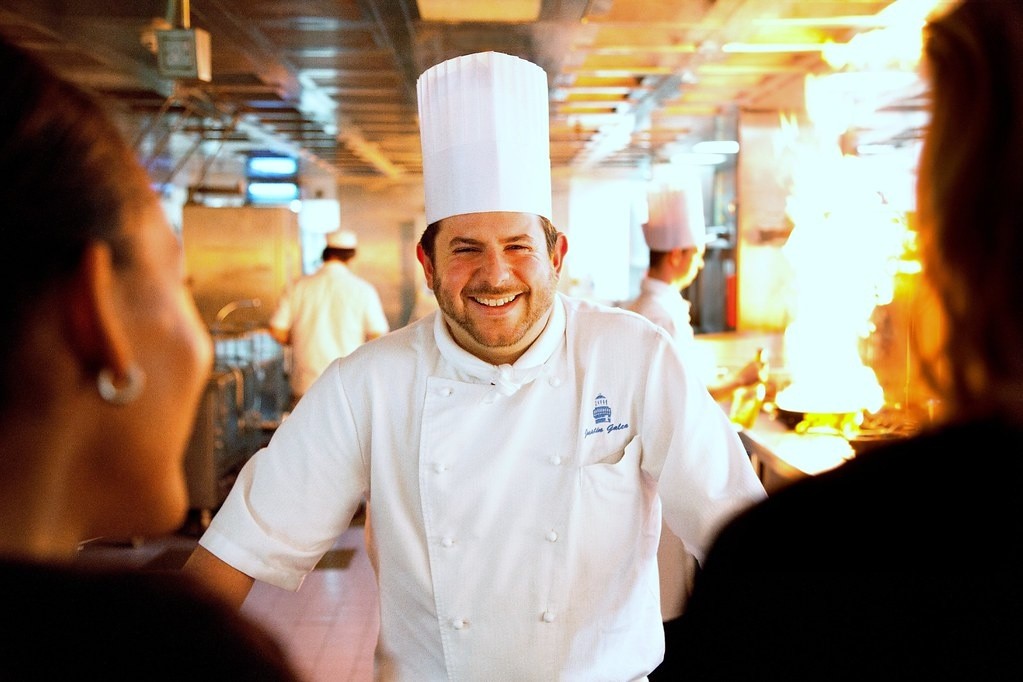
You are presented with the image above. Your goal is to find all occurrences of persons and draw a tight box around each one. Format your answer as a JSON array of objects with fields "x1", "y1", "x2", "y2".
[
  {"x1": 181, "y1": 188, "x2": 768, "y2": 681},
  {"x1": 645, "y1": 0, "x2": 1023, "y2": 682},
  {"x1": 269, "y1": 229, "x2": 390, "y2": 412},
  {"x1": 0, "y1": 30, "x2": 311, "y2": 682},
  {"x1": 622, "y1": 226, "x2": 791, "y2": 431}
]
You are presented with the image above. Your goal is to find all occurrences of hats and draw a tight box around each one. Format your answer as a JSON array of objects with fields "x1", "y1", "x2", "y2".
[
  {"x1": 416, "y1": 50, "x2": 552, "y2": 223},
  {"x1": 325, "y1": 228, "x2": 357, "y2": 249},
  {"x1": 641, "y1": 185, "x2": 706, "y2": 251}
]
